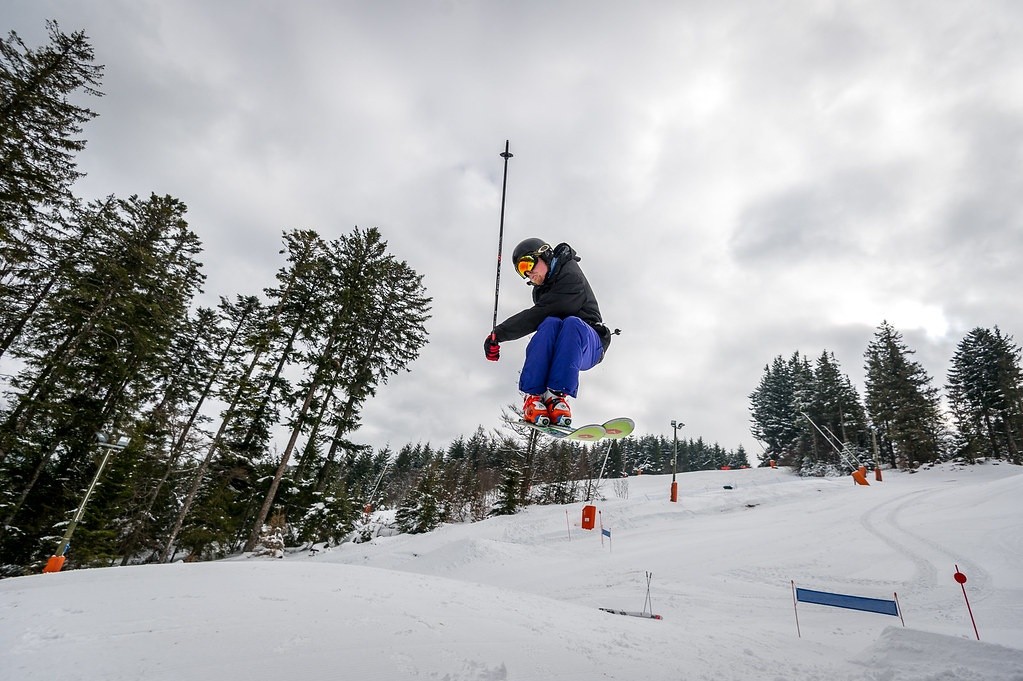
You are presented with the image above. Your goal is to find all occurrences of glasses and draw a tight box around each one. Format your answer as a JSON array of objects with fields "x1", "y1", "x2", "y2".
[{"x1": 515, "y1": 254, "x2": 538, "y2": 279}]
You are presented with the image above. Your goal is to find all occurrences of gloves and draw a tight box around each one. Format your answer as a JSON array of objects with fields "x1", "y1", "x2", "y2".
[{"x1": 484, "y1": 334, "x2": 500, "y2": 362}]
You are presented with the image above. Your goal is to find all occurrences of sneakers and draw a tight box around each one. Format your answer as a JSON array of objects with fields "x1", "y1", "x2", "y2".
[
  {"x1": 543, "y1": 387, "x2": 571, "y2": 427},
  {"x1": 522, "y1": 392, "x2": 550, "y2": 427}
]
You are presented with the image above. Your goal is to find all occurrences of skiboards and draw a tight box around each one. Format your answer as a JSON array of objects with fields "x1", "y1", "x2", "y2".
[
  {"x1": 598, "y1": 607, "x2": 663, "y2": 620},
  {"x1": 504, "y1": 416, "x2": 636, "y2": 441}
]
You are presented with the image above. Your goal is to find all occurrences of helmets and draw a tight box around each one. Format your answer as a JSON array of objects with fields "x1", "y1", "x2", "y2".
[{"x1": 512, "y1": 238, "x2": 554, "y2": 268}]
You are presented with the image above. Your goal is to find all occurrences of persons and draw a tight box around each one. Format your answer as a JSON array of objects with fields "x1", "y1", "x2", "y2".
[{"x1": 484, "y1": 238, "x2": 611, "y2": 426}]
